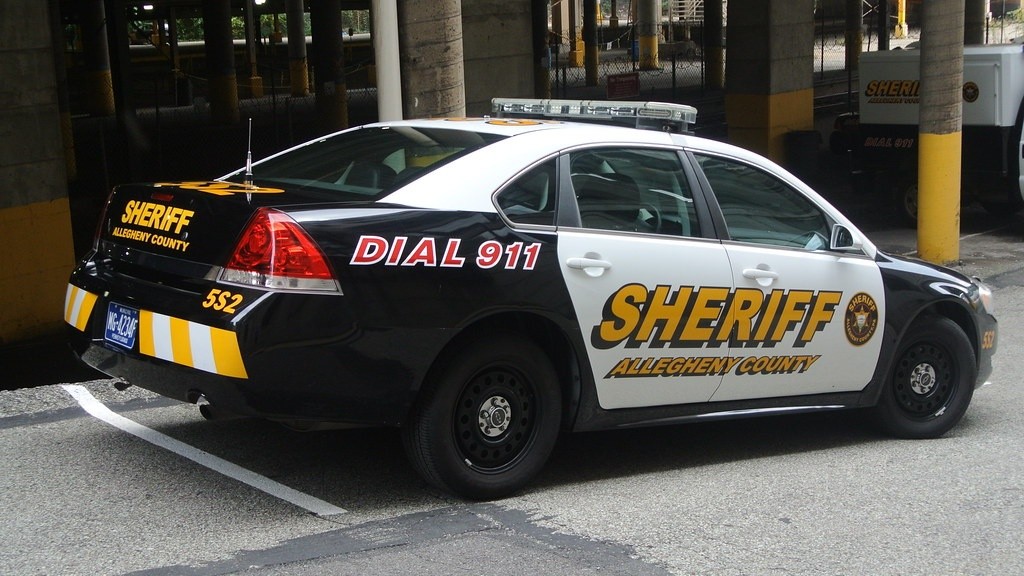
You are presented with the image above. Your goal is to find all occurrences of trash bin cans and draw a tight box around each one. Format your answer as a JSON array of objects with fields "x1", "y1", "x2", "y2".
[{"x1": 784, "y1": 129, "x2": 825, "y2": 195}]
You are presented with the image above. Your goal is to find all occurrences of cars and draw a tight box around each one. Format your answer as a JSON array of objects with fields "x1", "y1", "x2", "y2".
[{"x1": 63, "y1": 95, "x2": 998, "y2": 499}]
